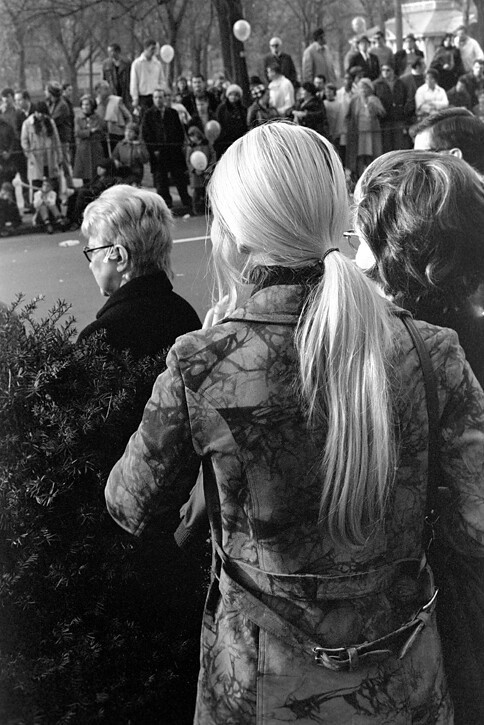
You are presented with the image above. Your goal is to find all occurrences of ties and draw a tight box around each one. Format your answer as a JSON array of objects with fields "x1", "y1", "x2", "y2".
[{"x1": 365, "y1": 52, "x2": 368, "y2": 62}]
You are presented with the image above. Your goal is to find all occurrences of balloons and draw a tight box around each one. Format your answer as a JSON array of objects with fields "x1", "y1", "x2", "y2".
[
  {"x1": 233, "y1": 18, "x2": 252, "y2": 43},
  {"x1": 189, "y1": 150, "x2": 210, "y2": 171},
  {"x1": 203, "y1": 119, "x2": 222, "y2": 144},
  {"x1": 160, "y1": 44, "x2": 175, "y2": 64},
  {"x1": 350, "y1": 16, "x2": 366, "y2": 34}
]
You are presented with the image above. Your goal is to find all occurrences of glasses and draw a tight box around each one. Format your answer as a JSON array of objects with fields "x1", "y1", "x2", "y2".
[
  {"x1": 83, "y1": 245, "x2": 130, "y2": 262},
  {"x1": 343, "y1": 229, "x2": 361, "y2": 252}
]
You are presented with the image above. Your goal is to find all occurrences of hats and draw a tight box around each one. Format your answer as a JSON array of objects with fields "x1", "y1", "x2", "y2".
[
  {"x1": 403, "y1": 33, "x2": 417, "y2": 41},
  {"x1": 356, "y1": 36, "x2": 371, "y2": 44},
  {"x1": 226, "y1": 83, "x2": 243, "y2": 98}
]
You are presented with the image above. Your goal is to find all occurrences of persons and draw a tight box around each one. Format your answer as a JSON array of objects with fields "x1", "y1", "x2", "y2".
[{"x1": 0, "y1": 24, "x2": 484, "y2": 725}]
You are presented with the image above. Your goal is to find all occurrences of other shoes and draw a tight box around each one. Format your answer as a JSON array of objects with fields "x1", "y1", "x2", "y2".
[
  {"x1": 47, "y1": 225, "x2": 54, "y2": 234},
  {"x1": 59, "y1": 221, "x2": 65, "y2": 233},
  {"x1": 185, "y1": 205, "x2": 197, "y2": 217}
]
